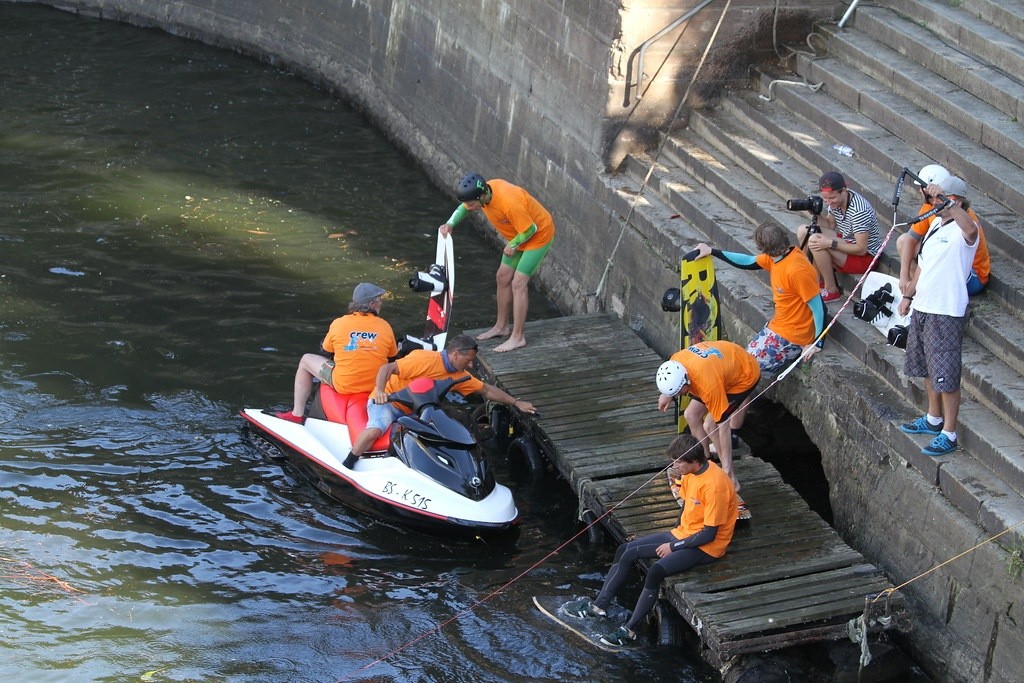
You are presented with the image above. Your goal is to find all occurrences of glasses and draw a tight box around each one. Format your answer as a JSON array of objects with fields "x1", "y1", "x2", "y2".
[
  {"x1": 464, "y1": 202, "x2": 475, "y2": 207},
  {"x1": 458, "y1": 344, "x2": 479, "y2": 353}
]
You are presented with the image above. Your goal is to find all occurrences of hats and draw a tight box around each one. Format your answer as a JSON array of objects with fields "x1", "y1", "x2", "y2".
[
  {"x1": 939, "y1": 176, "x2": 968, "y2": 199},
  {"x1": 352, "y1": 283, "x2": 386, "y2": 304},
  {"x1": 819, "y1": 172, "x2": 850, "y2": 192}
]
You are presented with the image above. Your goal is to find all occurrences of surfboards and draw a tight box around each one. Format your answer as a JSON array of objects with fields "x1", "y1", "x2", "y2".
[
  {"x1": 661, "y1": 248, "x2": 722, "y2": 437},
  {"x1": 395, "y1": 226, "x2": 456, "y2": 361},
  {"x1": 666, "y1": 466, "x2": 753, "y2": 520},
  {"x1": 852, "y1": 271, "x2": 916, "y2": 354}
]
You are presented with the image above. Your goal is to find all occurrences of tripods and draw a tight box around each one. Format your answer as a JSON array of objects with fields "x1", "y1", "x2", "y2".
[{"x1": 800, "y1": 215, "x2": 841, "y2": 290}]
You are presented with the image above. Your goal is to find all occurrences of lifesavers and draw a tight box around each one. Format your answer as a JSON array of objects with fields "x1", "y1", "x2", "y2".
[
  {"x1": 503, "y1": 437, "x2": 544, "y2": 478},
  {"x1": 646, "y1": 598, "x2": 679, "y2": 648},
  {"x1": 469, "y1": 402, "x2": 506, "y2": 434},
  {"x1": 576, "y1": 512, "x2": 600, "y2": 542}
]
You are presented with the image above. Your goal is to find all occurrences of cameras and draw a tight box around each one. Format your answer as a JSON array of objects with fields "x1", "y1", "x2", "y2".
[{"x1": 786, "y1": 195, "x2": 823, "y2": 216}]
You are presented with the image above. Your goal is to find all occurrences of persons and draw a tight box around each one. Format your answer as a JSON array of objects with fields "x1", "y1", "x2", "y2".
[
  {"x1": 564, "y1": 434, "x2": 738, "y2": 647},
  {"x1": 896, "y1": 165, "x2": 990, "y2": 456},
  {"x1": 656, "y1": 340, "x2": 761, "y2": 489},
  {"x1": 694, "y1": 221, "x2": 829, "y2": 453},
  {"x1": 341, "y1": 335, "x2": 537, "y2": 470},
  {"x1": 796, "y1": 171, "x2": 883, "y2": 304},
  {"x1": 441, "y1": 173, "x2": 556, "y2": 353},
  {"x1": 276, "y1": 283, "x2": 398, "y2": 425}
]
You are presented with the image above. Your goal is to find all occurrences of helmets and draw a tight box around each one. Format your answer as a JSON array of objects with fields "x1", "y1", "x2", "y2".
[
  {"x1": 457, "y1": 173, "x2": 488, "y2": 208},
  {"x1": 656, "y1": 361, "x2": 691, "y2": 397},
  {"x1": 914, "y1": 165, "x2": 951, "y2": 188}
]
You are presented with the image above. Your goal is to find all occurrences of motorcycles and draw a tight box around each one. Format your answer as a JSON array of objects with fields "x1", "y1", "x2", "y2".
[{"x1": 239, "y1": 340, "x2": 521, "y2": 545}]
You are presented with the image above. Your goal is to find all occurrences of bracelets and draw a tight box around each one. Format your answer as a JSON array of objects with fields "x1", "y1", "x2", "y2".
[
  {"x1": 903, "y1": 296, "x2": 914, "y2": 301},
  {"x1": 830, "y1": 240, "x2": 838, "y2": 250},
  {"x1": 944, "y1": 199, "x2": 955, "y2": 210},
  {"x1": 512, "y1": 399, "x2": 518, "y2": 407}
]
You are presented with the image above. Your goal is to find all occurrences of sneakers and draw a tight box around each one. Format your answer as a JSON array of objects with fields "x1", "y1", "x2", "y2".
[
  {"x1": 819, "y1": 278, "x2": 841, "y2": 304},
  {"x1": 900, "y1": 412, "x2": 943, "y2": 434},
  {"x1": 564, "y1": 601, "x2": 608, "y2": 621},
  {"x1": 921, "y1": 433, "x2": 958, "y2": 456},
  {"x1": 600, "y1": 623, "x2": 637, "y2": 647}
]
